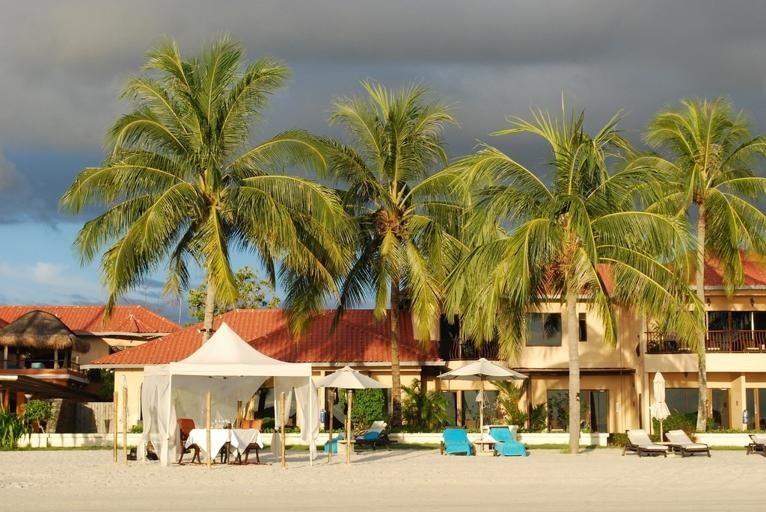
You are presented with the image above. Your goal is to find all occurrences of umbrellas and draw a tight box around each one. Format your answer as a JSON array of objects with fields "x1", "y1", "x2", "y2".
[
  {"x1": 315, "y1": 365, "x2": 393, "y2": 463},
  {"x1": 436, "y1": 357, "x2": 529, "y2": 452},
  {"x1": 650, "y1": 371, "x2": 671, "y2": 442}
]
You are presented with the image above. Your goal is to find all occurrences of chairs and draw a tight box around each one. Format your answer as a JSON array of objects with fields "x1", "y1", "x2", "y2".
[
  {"x1": 746, "y1": 435, "x2": 766, "y2": 457},
  {"x1": 622, "y1": 429, "x2": 668, "y2": 458},
  {"x1": 237, "y1": 420, "x2": 262, "y2": 463},
  {"x1": 177, "y1": 419, "x2": 202, "y2": 464},
  {"x1": 490, "y1": 426, "x2": 526, "y2": 457},
  {"x1": 355, "y1": 419, "x2": 392, "y2": 451},
  {"x1": 441, "y1": 427, "x2": 469, "y2": 456},
  {"x1": 665, "y1": 429, "x2": 711, "y2": 458},
  {"x1": 325, "y1": 432, "x2": 353, "y2": 454},
  {"x1": 221, "y1": 420, "x2": 253, "y2": 464}
]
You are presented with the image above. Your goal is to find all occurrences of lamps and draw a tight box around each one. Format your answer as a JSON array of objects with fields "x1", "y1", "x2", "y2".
[
  {"x1": 707, "y1": 298, "x2": 711, "y2": 305},
  {"x1": 750, "y1": 298, "x2": 755, "y2": 307}
]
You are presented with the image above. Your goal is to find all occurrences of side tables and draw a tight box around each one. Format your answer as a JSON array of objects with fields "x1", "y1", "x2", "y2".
[{"x1": 472, "y1": 438, "x2": 497, "y2": 456}]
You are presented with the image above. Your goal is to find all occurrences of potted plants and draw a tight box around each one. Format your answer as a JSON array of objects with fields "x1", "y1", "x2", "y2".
[{"x1": 21, "y1": 400, "x2": 52, "y2": 432}]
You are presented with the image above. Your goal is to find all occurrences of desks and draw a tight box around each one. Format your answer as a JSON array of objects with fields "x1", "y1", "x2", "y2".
[{"x1": 185, "y1": 428, "x2": 264, "y2": 465}]
